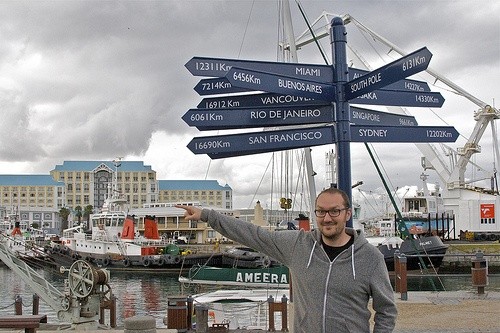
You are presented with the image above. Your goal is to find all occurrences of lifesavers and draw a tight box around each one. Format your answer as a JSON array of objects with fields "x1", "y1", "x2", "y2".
[
  {"x1": 263, "y1": 258, "x2": 271, "y2": 267},
  {"x1": 85, "y1": 256, "x2": 90, "y2": 262},
  {"x1": 480, "y1": 233, "x2": 487, "y2": 240},
  {"x1": 71, "y1": 254, "x2": 77, "y2": 260},
  {"x1": 60, "y1": 265, "x2": 65, "y2": 273},
  {"x1": 103, "y1": 259, "x2": 111, "y2": 266},
  {"x1": 157, "y1": 250, "x2": 162, "y2": 254},
  {"x1": 117, "y1": 232, "x2": 123, "y2": 238},
  {"x1": 158, "y1": 258, "x2": 166, "y2": 266},
  {"x1": 44, "y1": 244, "x2": 52, "y2": 250},
  {"x1": 173, "y1": 256, "x2": 181, "y2": 264},
  {"x1": 122, "y1": 259, "x2": 130, "y2": 267},
  {"x1": 489, "y1": 233, "x2": 496, "y2": 241},
  {"x1": 143, "y1": 258, "x2": 151, "y2": 267},
  {"x1": 59, "y1": 249, "x2": 64, "y2": 256}
]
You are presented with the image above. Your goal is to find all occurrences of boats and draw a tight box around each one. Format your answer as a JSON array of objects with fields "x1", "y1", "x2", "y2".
[{"x1": 0, "y1": 0, "x2": 500, "y2": 309}]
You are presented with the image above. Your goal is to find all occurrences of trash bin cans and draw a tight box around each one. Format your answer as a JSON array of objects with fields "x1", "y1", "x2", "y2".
[
  {"x1": 167, "y1": 296, "x2": 194, "y2": 333},
  {"x1": 470, "y1": 258, "x2": 489, "y2": 287}
]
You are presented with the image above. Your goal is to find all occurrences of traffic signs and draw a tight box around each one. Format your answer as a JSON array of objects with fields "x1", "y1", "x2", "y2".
[
  {"x1": 181, "y1": 104, "x2": 335, "y2": 127},
  {"x1": 196, "y1": 91, "x2": 332, "y2": 109},
  {"x1": 350, "y1": 124, "x2": 460, "y2": 143},
  {"x1": 196, "y1": 122, "x2": 286, "y2": 131},
  {"x1": 348, "y1": 105, "x2": 419, "y2": 126},
  {"x1": 206, "y1": 147, "x2": 293, "y2": 160},
  {"x1": 184, "y1": 56, "x2": 335, "y2": 83},
  {"x1": 344, "y1": 46, "x2": 433, "y2": 103},
  {"x1": 225, "y1": 66, "x2": 335, "y2": 102},
  {"x1": 348, "y1": 88, "x2": 445, "y2": 108},
  {"x1": 185, "y1": 125, "x2": 335, "y2": 155},
  {"x1": 192, "y1": 77, "x2": 261, "y2": 96},
  {"x1": 348, "y1": 67, "x2": 431, "y2": 92}
]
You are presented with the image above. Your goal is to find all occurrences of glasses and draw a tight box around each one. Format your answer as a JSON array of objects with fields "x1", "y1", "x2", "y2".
[{"x1": 314, "y1": 207, "x2": 348, "y2": 218}]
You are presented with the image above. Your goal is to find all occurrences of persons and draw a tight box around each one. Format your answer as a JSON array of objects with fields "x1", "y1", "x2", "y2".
[{"x1": 173, "y1": 188, "x2": 398, "y2": 333}]
